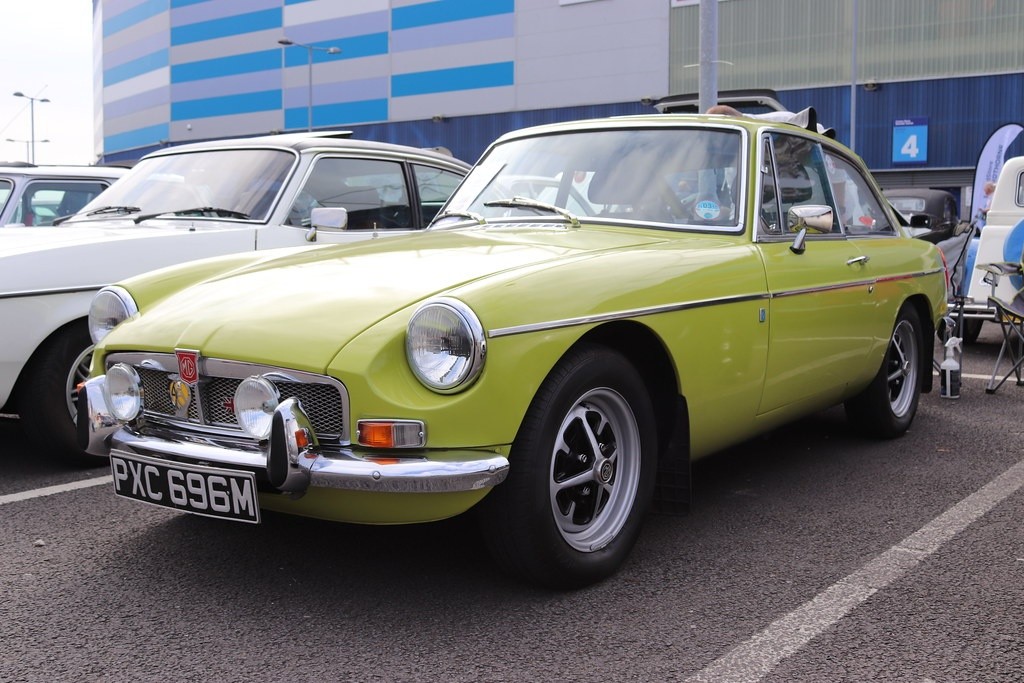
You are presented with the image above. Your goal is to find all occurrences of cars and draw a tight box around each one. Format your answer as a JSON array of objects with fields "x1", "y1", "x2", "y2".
[
  {"x1": 74, "y1": 111, "x2": 950, "y2": 588},
  {"x1": 0, "y1": 136, "x2": 543, "y2": 469},
  {"x1": 882, "y1": 188, "x2": 960, "y2": 241}
]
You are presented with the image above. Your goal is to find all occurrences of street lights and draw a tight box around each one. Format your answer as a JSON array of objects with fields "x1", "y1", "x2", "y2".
[
  {"x1": 7, "y1": 138, "x2": 50, "y2": 167},
  {"x1": 278, "y1": 36, "x2": 343, "y2": 134},
  {"x1": 14, "y1": 92, "x2": 51, "y2": 166}
]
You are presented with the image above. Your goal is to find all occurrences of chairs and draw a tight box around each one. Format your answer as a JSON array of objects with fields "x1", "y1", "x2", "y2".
[
  {"x1": 976, "y1": 249, "x2": 1024, "y2": 391},
  {"x1": 587, "y1": 141, "x2": 676, "y2": 226},
  {"x1": 761, "y1": 157, "x2": 814, "y2": 223},
  {"x1": 212, "y1": 173, "x2": 305, "y2": 226},
  {"x1": 321, "y1": 184, "x2": 388, "y2": 229},
  {"x1": 58, "y1": 190, "x2": 88, "y2": 216}
]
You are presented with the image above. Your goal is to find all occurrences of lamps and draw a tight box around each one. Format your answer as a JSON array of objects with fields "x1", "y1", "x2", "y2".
[
  {"x1": 641, "y1": 98, "x2": 652, "y2": 106},
  {"x1": 432, "y1": 115, "x2": 443, "y2": 124},
  {"x1": 864, "y1": 81, "x2": 876, "y2": 91}
]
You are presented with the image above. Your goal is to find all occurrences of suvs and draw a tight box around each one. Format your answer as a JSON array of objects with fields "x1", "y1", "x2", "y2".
[{"x1": 0, "y1": 161, "x2": 134, "y2": 230}]
